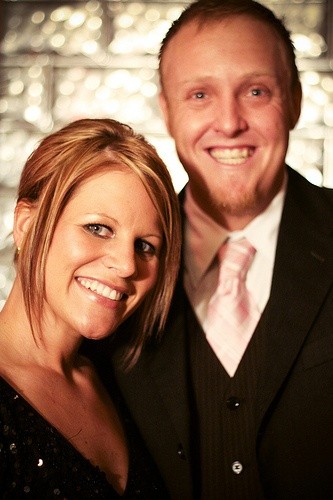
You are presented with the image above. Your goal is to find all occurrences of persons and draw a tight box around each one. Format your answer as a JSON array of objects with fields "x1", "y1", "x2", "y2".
[
  {"x1": 95, "y1": 0, "x2": 333, "y2": 500},
  {"x1": 0, "y1": 116, "x2": 183, "y2": 500}
]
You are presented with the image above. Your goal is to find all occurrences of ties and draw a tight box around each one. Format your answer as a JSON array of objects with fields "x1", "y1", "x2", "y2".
[{"x1": 202, "y1": 236, "x2": 254, "y2": 377}]
